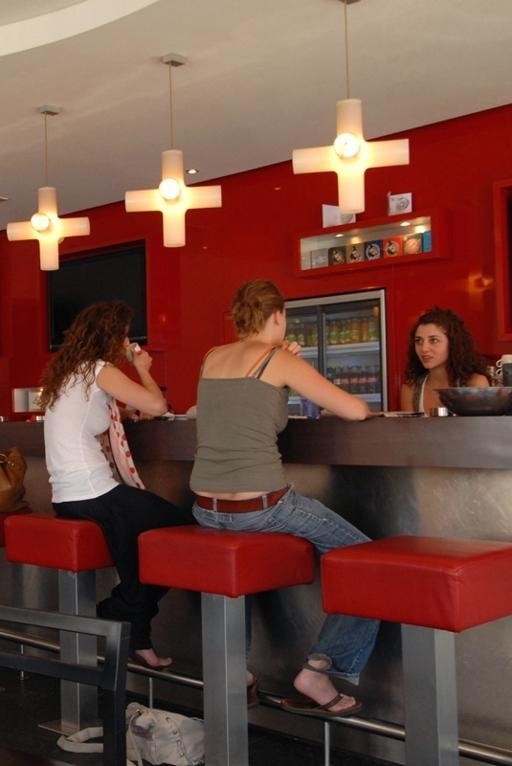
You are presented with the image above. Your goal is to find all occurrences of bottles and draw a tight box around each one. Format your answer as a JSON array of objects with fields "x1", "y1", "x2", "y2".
[{"x1": 288, "y1": 308, "x2": 381, "y2": 397}]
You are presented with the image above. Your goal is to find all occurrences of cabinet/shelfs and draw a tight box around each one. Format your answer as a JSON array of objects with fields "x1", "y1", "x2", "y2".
[{"x1": 293, "y1": 207, "x2": 451, "y2": 278}]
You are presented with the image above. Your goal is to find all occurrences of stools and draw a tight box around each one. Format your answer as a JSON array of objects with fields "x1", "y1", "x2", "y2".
[
  {"x1": 321, "y1": 536, "x2": 512, "y2": 766},
  {"x1": 4, "y1": 512, "x2": 114, "y2": 737},
  {"x1": 139, "y1": 525, "x2": 313, "y2": 766}
]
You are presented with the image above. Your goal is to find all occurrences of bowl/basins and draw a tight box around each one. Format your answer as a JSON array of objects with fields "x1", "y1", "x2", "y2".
[{"x1": 433, "y1": 385, "x2": 512, "y2": 416}]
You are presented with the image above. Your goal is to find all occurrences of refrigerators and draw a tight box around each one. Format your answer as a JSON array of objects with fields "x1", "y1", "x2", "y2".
[{"x1": 280, "y1": 288, "x2": 389, "y2": 418}]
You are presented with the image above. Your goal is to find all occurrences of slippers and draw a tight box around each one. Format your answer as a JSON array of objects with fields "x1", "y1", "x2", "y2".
[
  {"x1": 282, "y1": 673, "x2": 363, "y2": 721},
  {"x1": 127, "y1": 642, "x2": 174, "y2": 675},
  {"x1": 247, "y1": 674, "x2": 260, "y2": 704}
]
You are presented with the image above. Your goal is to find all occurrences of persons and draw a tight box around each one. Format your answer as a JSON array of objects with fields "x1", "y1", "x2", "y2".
[
  {"x1": 34, "y1": 299, "x2": 197, "y2": 670},
  {"x1": 400, "y1": 307, "x2": 490, "y2": 417},
  {"x1": 188, "y1": 280, "x2": 384, "y2": 717}
]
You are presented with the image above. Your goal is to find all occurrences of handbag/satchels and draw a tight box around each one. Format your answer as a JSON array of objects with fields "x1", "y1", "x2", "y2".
[
  {"x1": 0, "y1": 445, "x2": 32, "y2": 515},
  {"x1": 125, "y1": 701, "x2": 204, "y2": 766}
]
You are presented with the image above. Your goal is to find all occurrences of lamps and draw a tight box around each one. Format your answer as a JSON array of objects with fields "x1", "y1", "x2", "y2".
[
  {"x1": 125, "y1": 53, "x2": 221, "y2": 247},
  {"x1": 7, "y1": 107, "x2": 90, "y2": 271},
  {"x1": 292, "y1": 0, "x2": 410, "y2": 214}
]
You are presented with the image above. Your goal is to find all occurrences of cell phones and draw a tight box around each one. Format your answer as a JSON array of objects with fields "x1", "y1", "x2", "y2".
[{"x1": 384, "y1": 411, "x2": 425, "y2": 417}]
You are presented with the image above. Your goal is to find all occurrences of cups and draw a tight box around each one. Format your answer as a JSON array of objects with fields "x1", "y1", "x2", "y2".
[
  {"x1": 124, "y1": 341, "x2": 144, "y2": 362},
  {"x1": 486, "y1": 353, "x2": 511, "y2": 386}
]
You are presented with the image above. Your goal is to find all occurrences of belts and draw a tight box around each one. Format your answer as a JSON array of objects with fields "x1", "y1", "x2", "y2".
[{"x1": 195, "y1": 486, "x2": 290, "y2": 514}]
